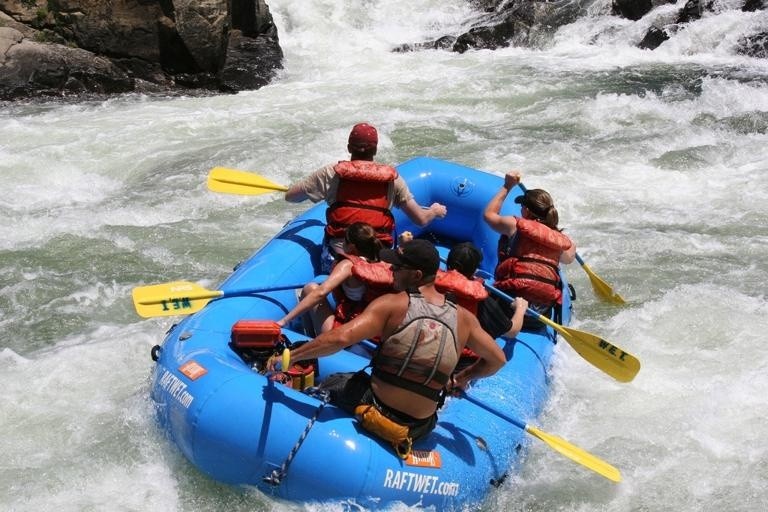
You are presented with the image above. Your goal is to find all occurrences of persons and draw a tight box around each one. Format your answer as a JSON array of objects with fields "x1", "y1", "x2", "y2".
[
  {"x1": 486, "y1": 171, "x2": 576, "y2": 330},
  {"x1": 285, "y1": 124, "x2": 445, "y2": 273},
  {"x1": 265, "y1": 240, "x2": 508, "y2": 441},
  {"x1": 276, "y1": 223, "x2": 395, "y2": 337},
  {"x1": 436, "y1": 243, "x2": 528, "y2": 336}
]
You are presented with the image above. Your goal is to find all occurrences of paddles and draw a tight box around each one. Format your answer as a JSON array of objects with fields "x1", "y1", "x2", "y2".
[
  {"x1": 513, "y1": 173, "x2": 627, "y2": 304},
  {"x1": 132, "y1": 280, "x2": 326, "y2": 318},
  {"x1": 206, "y1": 165, "x2": 432, "y2": 211},
  {"x1": 360, "y1": 338, "x2": 621, "y2": 483},
  {"x1": 439, "y1": 255, "x2": 641, "y2": 383}
]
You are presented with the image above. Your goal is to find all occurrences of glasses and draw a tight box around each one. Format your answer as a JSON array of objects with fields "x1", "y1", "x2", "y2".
[{"x1": 389, "y1": 262, "x2": 406, "y2": 271}]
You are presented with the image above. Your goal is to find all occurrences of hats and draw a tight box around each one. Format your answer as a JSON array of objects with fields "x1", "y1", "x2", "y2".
[
  {"x1": 348, "y1": 124, "x2": 379, "y2": 151},
  {"x1": 380, "y1": 239, "x2": 441, "y2": 275},
  {"x1": 514, "y1": 197, "x2": 548, "y2": 217},
  {"x1": 448, "y1": 242, "x2": 484, "y2": 266}
]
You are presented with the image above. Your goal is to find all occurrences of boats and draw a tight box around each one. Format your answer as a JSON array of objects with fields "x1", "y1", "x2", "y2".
[{"x1": 149, "y1": 158, "x2": 577, "y2": 512}]
